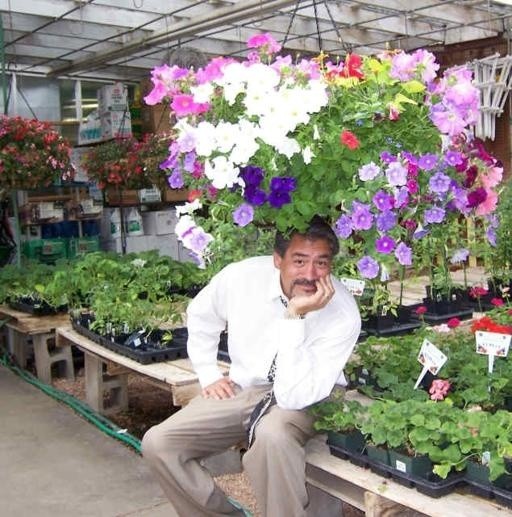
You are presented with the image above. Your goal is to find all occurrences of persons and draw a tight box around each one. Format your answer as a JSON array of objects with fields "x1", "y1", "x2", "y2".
[{"x1": 141, "y1": 218, "x2": 361, "y2": 517}]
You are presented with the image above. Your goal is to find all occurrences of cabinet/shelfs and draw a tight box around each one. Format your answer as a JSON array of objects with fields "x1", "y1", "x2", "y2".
[
  {"x1": 178, "y1": 242, "x2": 200, "y2": 265},
  {"x1": 100, "y1": 236, "x2": 179, "y2": 261},
  {"x1": 139, "y1": 210, "x2": 179, "y2": 235}
]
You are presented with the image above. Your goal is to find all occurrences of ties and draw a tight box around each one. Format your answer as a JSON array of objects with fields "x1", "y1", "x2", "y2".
[{"x1": 246, "y1": 296, "x2": 305, "y2": 451}]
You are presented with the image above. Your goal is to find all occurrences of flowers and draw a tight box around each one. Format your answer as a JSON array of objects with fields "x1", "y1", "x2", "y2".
[{"x1": 143, "y1": 32, "x2": 512, "y2": 342}]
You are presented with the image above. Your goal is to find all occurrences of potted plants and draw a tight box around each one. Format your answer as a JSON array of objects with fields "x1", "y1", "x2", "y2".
[
  {"x1": 0, "y1": 257, "x2": 74, "y2": 316},
  {"x1": 304, "y1": 397, "x2": 512, "y2": 509},
  {"x1": 176, "y1": 261, "x2": 218, "y2": 298},
  {"x1": 343, "y1": 305, "x2": 512, "y2": 414},
  {"x1": 79, "y1": 137, "x2": 163, "y2": 205},
  {"x1": 120, "y1": 249, "x2": 184, "y2": 300},
  {"x1": 127, "y1": 132, "x2": 188, "y2": 202},
  {"x1": 0, "y1": 60, "x2": 77, "y2": 192},
  {"x1": 57, "y1": 250, "x2": 145, "y2": 309},
  {"x1": 69, "y1": 259, "x2": 232, "y2": 365}
]
